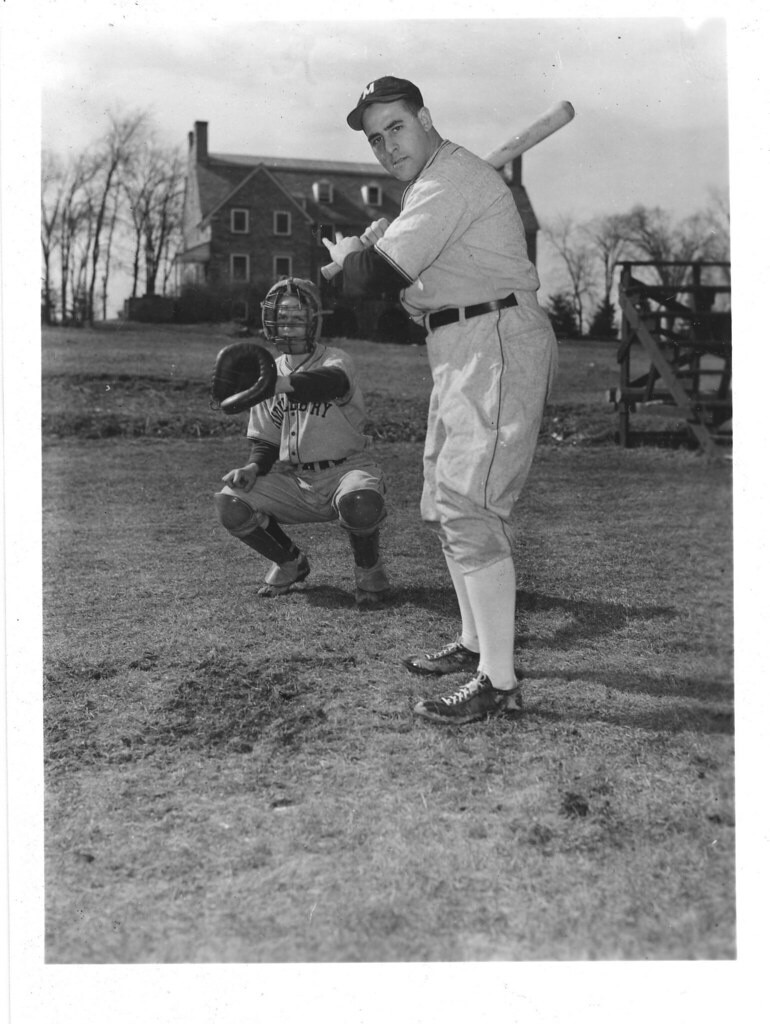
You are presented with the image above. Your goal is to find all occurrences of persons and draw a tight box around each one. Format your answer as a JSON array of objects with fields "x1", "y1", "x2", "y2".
[
  {"x1": 321, "y1": 75, "x2": 557, "y2": 726},
  {"x1": 211, "y1": 275, "x2": 397, "y2": 613}
]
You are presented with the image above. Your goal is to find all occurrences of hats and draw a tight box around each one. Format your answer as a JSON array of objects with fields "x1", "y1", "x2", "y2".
[{"x1": 347, "y1": 76, "x2": 422, "y2": 131}]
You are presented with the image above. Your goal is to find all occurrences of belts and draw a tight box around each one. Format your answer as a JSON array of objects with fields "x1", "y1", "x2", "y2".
[
  {"x1": 424, "y1": 293, "x2": 518, "y2": 331},
  {"x1": 302, "y1": 456, "x2": 346, "y2": 470}
]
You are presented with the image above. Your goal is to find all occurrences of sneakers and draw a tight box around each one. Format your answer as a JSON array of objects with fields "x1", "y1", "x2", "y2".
[
  {"x1": 258, "y1": 553, "x2": 310, "y2": 598},
  {"x1": 355, "y1": 587, "x2": 385, "y2": 610},
  {"x1": 415, "y1": 671, "x2": 522, "y2": 724},
  {"x1": 396, "y1": 633, "x2": 480, "y2": 675}
]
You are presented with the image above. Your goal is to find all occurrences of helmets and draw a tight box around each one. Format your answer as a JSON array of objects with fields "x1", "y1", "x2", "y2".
[{"x1": 261, "y1": 278, "x2": 324, "y2": 355}]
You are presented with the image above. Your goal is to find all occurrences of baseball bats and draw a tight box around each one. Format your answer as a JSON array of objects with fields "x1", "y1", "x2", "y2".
[{"x1": 319, "y1": 98, "x2": 576, "y2": 281}]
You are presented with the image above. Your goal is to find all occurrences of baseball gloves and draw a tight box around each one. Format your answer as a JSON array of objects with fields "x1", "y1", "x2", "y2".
[{"x1": 206, "y1": 341, "x2": 279, "y2": 416}]
